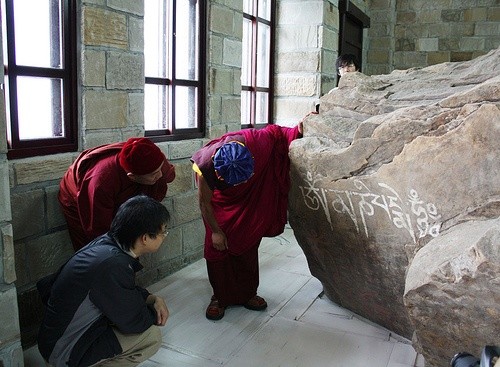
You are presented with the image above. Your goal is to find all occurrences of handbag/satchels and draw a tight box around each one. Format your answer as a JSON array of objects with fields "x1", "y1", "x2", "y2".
[{"x1": 35, "y1": 273, "x2": 57, "y2": 307}]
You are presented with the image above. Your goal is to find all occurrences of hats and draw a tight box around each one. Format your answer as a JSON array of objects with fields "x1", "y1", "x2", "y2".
[
  {"x1": 213, "y1": 140, "x2": 255, "y2": 187},
  {"x1": 118, "y1": 137, "x2": 163, "y2": 176}
]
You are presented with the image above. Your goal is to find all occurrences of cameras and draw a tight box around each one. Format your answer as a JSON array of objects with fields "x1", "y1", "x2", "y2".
[{"x1": 450, "y1": 345, "x2": 500, "y2": 367}]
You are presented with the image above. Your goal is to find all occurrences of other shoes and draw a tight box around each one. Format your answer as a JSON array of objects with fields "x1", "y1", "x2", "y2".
[
  {"x1": 242, "y1": 295, "x2": 267, "y2": 310},
  {"x1": 205, "y1": 294, "x2": 228, "y2": 321}
]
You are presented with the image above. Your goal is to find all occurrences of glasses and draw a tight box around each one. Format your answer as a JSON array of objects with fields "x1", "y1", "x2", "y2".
[
  {"x1": 337, "y1": 65, "x2": 356, "y2": 69},
  {"x1": 150, "y1": 231, "x2": 169, "y2": 240}
]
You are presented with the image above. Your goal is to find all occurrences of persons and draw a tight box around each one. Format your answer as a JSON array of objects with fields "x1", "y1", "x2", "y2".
[
  {"x1": 58, "y1": 137, "x2": 176, "y2": 252},
  {"x1": 335, "y1": 54, "x2": 360, "y2": 75},
  {"x1": 190, "y1": 112, "x2": 318, "y2": 321},
  {"x1": 37, "y1": 194, "x2": 171, "y2": 367}
]
